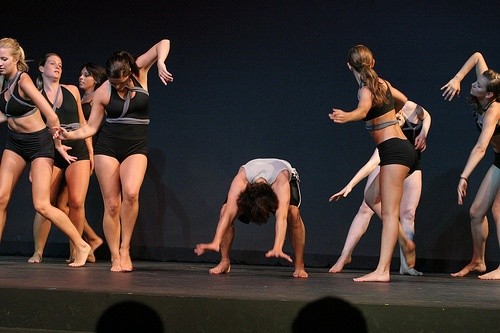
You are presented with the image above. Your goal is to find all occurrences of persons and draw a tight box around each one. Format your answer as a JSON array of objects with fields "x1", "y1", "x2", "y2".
[
  {"x1": 50, "y1": 40, "x2": 173, "y2": 272},
  {"x1": 193, "y1": 159, "x2": 310, "y2": 279},
  {"x1": 440, "y1": 51, "x2": 500, "y2": 280},
  {"x1": 0, "y1": 38, "x2": 107, "y2": 268},
  {"x1": 326, "y1": 44, "x2": 432, "y2": 281}
]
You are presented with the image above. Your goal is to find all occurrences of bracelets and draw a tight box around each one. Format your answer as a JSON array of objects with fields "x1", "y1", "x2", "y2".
[{"x1": 459, "y1": 177, "x2": 469, "y2": 184}]
